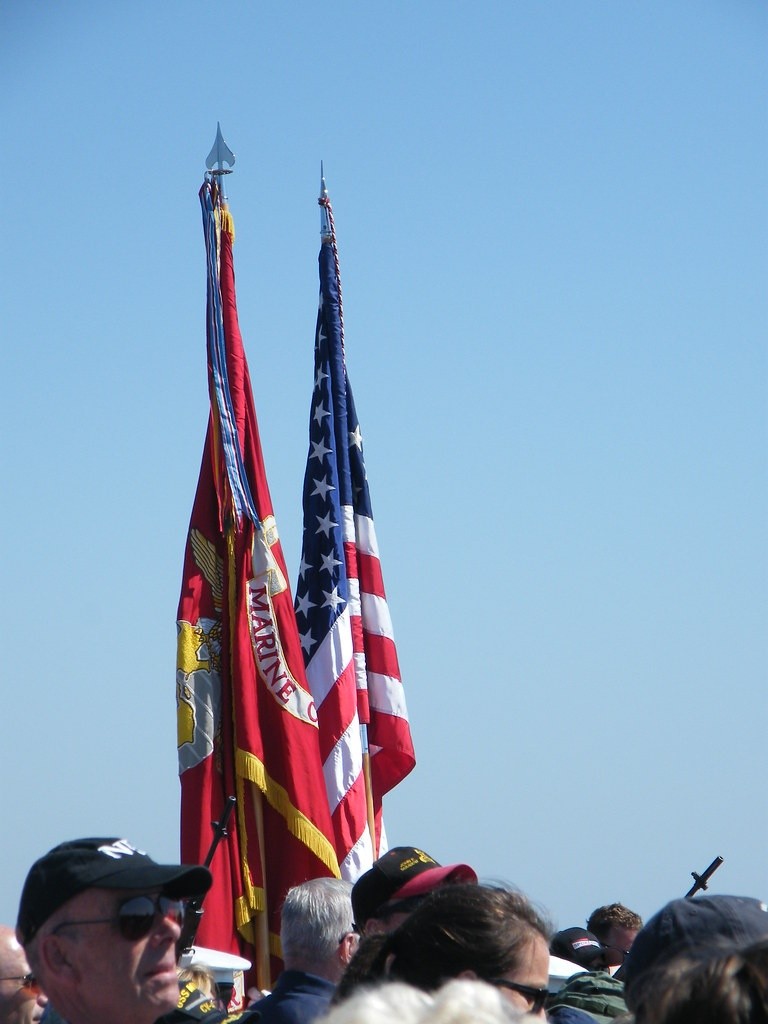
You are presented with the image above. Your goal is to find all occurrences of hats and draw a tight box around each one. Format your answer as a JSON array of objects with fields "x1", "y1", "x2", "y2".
[
  {"x1": 16, "y1": 839, "x2": 213, "y2": 946},
  {"x1": 351, "y1": 847, "x2": 478, "y2": 932},
  {"x1": 546, "y1": 956, "x2": 589, "y2": 994},
  {"x1": 176, "y1": 980, "x2": 224, "y2": 1024},
  {"x1": 622, "y1": 894, "x2": 768, "y2": 982},
  {"x1": 549, "y1": 927, "x2": 624, "y2": 967},
  {"x1": 177, "y1": 945, "x2": 252, "y2": 983}
]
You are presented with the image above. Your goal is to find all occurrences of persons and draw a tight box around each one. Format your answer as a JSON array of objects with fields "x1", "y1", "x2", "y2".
[{"x1": 0, "y1": 835, "x2": 767, "y2": 1024}]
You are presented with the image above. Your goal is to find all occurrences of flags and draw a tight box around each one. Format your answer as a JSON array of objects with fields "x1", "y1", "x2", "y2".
[{"x1": 176, "y1": 182, "x2": 416, "y2": 1022}]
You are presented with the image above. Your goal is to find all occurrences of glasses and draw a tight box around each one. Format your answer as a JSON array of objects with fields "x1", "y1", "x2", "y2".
[
  {"x1": 492, "y1": 978, "x2": 549, "y2": 1014},
  {"x1": 380, "y1": 892, "x2": 430, "y2": 914},
  {"x1": 0, "y1": 971, "x2": 42, "y2": 996},
  {"x1": 52, "y1": 892, "x2": 186, "y2": 941},
  {"x1": 582, "y1": 965, "x2": 610, "y2": 976},
  {"x1": 600, "y1": 943, "x2": 629, "y2": 956}
]
